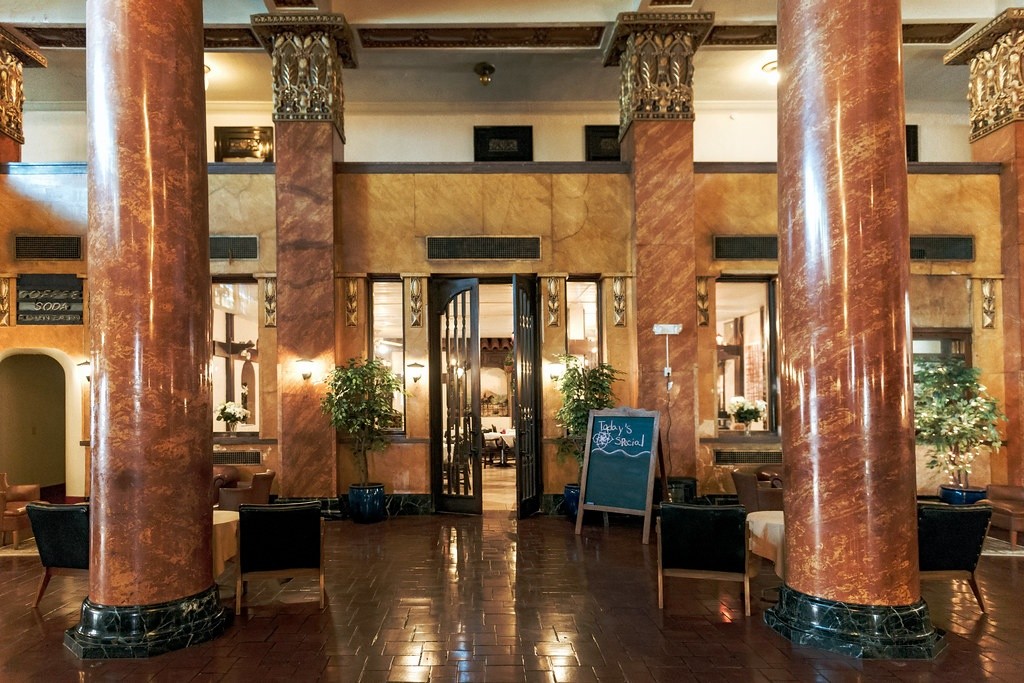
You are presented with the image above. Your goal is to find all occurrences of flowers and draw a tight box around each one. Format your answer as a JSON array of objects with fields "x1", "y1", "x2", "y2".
[
  {"x1": 240, "y1": 382, "x2": 250, "y2": 412},
  {"x1": 723, "y1": 393, "x2": 768, "y2": 428},
  {"x1": 212, "y1": 400, "x2": 251, "y2": 431}
]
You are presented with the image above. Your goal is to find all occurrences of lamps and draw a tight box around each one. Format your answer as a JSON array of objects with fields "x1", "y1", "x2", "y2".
[
  {"x1": 293, "y1": 357, "x2": 315, "y2": 382},
  {"x1": 546, "y1": 359, "x2": 566, "y2": 384},
  {"x1": 405, "y1": 359, "x2": 425, "y2": 384}
]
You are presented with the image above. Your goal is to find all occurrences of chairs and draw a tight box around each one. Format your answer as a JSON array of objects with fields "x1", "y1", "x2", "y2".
[
  {"x1": 0, "y1": 462, "x2": 328, "y2": 618},
  {"x1": 915, "y1": 499, "x2": 998, "y2": 619},
  {"x1": 972, "y1": 483, "x2": 1024, "y2": 554},
  {"x1": 652, "y1": 462, "x2": 785, "y2": 619}
]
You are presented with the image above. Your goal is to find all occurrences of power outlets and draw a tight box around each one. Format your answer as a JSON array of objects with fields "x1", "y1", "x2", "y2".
[{"x1": 663, "y1": 365, "x2": 673, "y2": 378}]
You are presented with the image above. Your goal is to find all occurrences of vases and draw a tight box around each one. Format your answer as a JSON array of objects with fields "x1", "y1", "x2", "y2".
[
  {"x1": 742, "y1": 419, "x2": 753, "y2": 444},
  {"x1": 227, "y1": 422, "x2": 239, "y2": 440}
]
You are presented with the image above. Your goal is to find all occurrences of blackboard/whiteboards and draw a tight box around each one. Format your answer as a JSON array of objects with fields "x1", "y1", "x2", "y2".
[{"x1": 581, "y1": 410, "x2": 659, "y2": 516}]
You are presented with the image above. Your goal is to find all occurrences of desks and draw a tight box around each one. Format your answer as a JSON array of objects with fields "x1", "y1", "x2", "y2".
[
  {"x1": 484, "y1": 431, "x2": 516, "y2": 472},
  {"x1": 212, "y1": 508, "x2": 241, "y2": 584},
  {"x1": 746, "y1": 507, "x2": 789, "y2": 604}
]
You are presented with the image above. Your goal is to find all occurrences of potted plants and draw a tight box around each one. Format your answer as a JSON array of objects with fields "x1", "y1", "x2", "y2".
[
  {"x1": 910, "y1": 348, "x2": 1011, "y2": 508},
  {"x1": 315, "y1": 354, "x2": 416, "y2": 526},
  {"x1": 541, "y1": 350, "x2": 629, "y2": 526}
]
